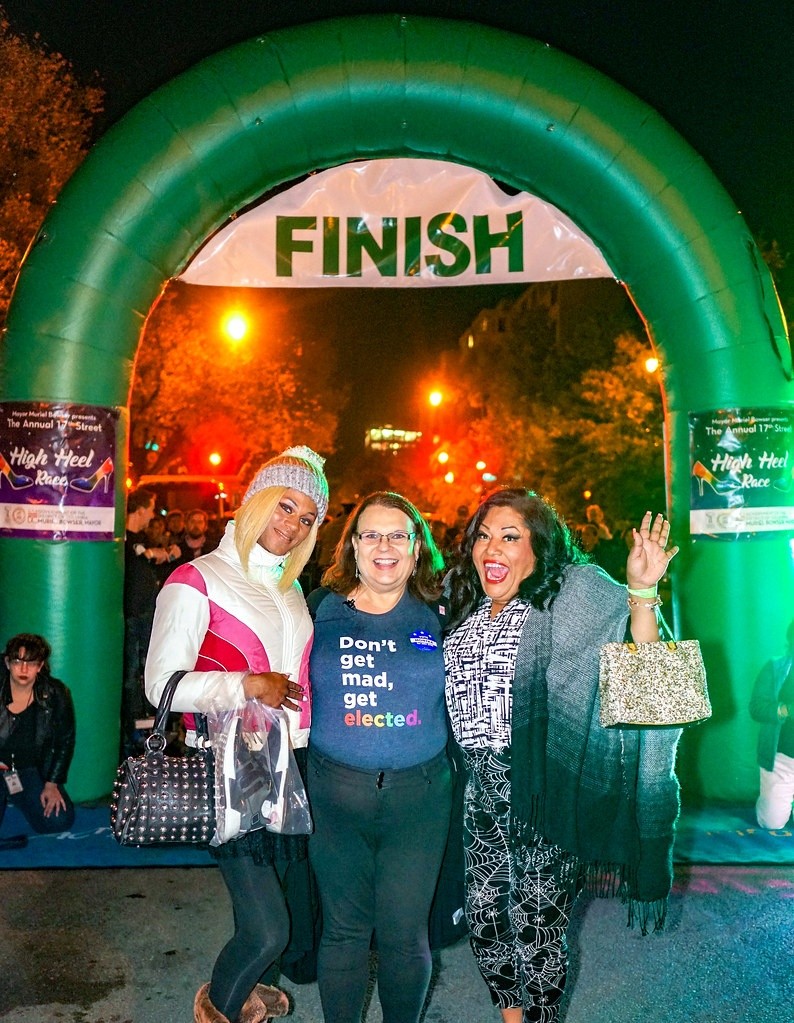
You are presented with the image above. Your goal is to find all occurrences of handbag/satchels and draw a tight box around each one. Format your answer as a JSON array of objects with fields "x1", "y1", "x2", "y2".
[
  {"x1": 599, "y1": 614, "x2": 712, "y2": 730},
  {"x1": 112, "y1": 669, "x2": 216, "y2": 847}
]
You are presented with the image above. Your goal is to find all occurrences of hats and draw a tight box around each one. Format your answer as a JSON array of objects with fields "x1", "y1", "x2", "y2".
[{"x1": 241, "y1": 444, "x2": 330, "y2": 526}]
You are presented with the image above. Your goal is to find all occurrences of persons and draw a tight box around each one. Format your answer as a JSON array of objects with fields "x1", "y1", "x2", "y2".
[
  {"x1": 749, "y1": 619, "x2": 794, "y2": 828},
  {"x1": 303, "y1": 489, "x2": 462, "y2": 1023},
  {"x1": 0, "y1": 633, "x2": 81, "y2": 834},
  {"x1": 442, "y1": 487, "x2": 685, "y2": 1022},
  {"x1": 138, "y1": 445, "x2": 330, "y2": 1023},
  {"x1": 114, "y1": 473, "x2": 673, "y2": 755}
]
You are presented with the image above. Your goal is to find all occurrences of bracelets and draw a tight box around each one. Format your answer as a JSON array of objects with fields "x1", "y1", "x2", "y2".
[{"x1": 624, "y1": 585, "x2": 665, "y2": 610}]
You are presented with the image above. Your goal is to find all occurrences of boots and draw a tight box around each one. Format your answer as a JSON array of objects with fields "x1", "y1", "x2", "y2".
[{"x1": 193, "y1": 978, "x2": 289, "y2": 1023}]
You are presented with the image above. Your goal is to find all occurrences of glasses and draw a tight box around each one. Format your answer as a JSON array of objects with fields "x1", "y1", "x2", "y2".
[
  {"x1": 10, "y1": 657, "x2": 39, "y2": 668},
  {"x1": 355, "y1": 531, "x2": 418, "y2": 545}
]
